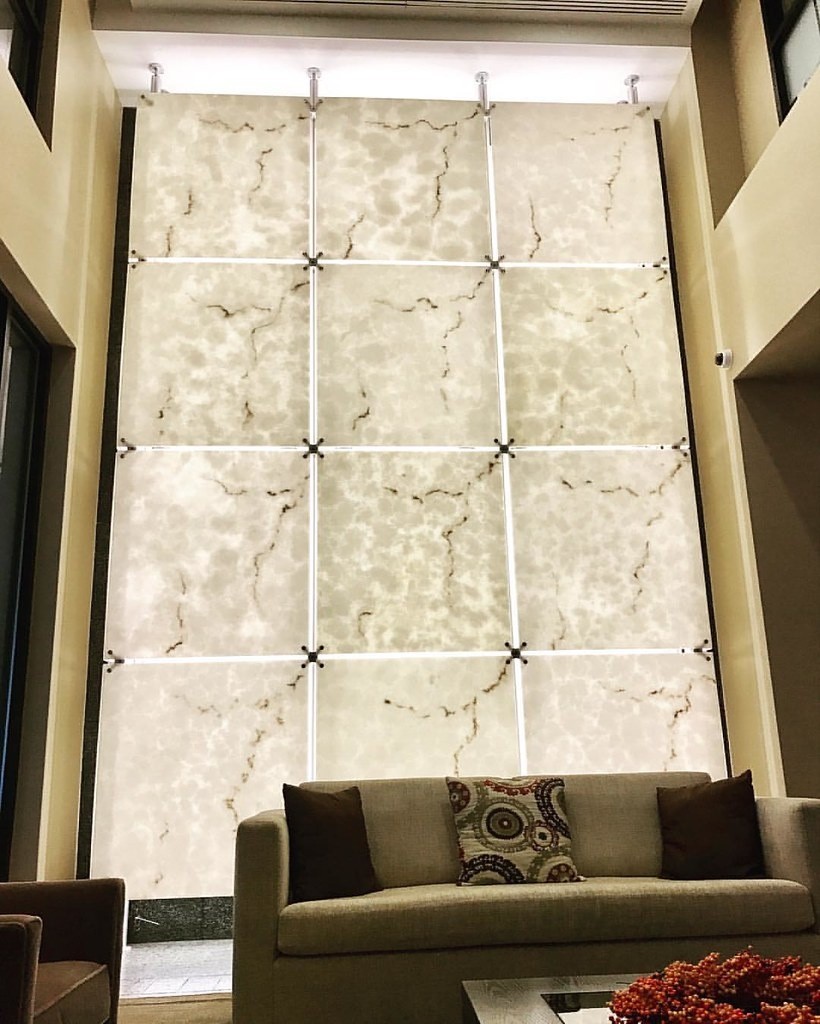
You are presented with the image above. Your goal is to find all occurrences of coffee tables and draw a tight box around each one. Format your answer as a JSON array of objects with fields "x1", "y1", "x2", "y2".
[{"x1": 459, "y1": 972, "x2": 657, "y2": 1024}]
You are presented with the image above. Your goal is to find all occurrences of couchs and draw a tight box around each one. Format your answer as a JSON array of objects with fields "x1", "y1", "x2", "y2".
[
  {"x1": 231, "y1": 769, "x2": 820, "y2": 1024},
  {"x1": 0, "y1": 879, "x2": 125, "y2": 1024}
]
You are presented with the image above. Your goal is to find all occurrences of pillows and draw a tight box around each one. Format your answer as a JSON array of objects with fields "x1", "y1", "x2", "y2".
[
  {"x1": 445, "y1": 776, "x2": 586, "y2": 886},
  {"x1": 656, "y1": 769, "x2": 767, "y2": 879},
  {"x1": 282, "y1": 784, "x2": 382, "y2": 904}
]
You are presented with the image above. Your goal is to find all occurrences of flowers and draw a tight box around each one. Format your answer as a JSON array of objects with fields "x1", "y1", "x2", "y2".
[{"x1": 605, "y1": 948, "x2": 820, "y2": 1024}]
